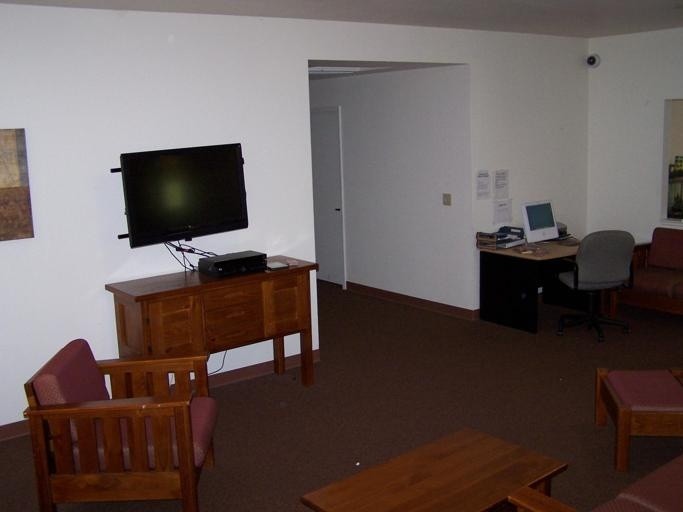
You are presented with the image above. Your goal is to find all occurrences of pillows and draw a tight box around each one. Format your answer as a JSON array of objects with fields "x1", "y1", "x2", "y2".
[
  {"x1": 31, "y1": 339, "x2": 112, "y2": 450},
  {"x1": 648, "y1": 227, "x2": 683, "y2": 268}
]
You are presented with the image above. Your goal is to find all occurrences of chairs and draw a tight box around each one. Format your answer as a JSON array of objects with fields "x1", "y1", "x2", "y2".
[
  {"x1": 22, "y1": 340, "x2": 220, "y2": 512},
  {"x1": 603, "y1": 242, "x2": 683, "y2": 323},
  {"x1": 554, "y1": 229, "x2": 635, "y2": 343}
]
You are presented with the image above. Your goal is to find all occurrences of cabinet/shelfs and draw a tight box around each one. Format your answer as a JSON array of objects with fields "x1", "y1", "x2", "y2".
[
  {"x1": 104, "y1": 257, "x2": 319, "y2": 397},
  {"x1": 478, "y1": 235, "x2": 580, "y2": 333}
]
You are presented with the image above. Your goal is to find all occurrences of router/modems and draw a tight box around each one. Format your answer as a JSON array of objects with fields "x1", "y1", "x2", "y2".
[{"x1": 198, "y1": 251, "x2": 267, "y2": 277}]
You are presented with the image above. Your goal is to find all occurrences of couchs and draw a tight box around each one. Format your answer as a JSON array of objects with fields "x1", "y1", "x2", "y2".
[{"x1": 506, "y1": 454, "x2": 683, "y2": 511}]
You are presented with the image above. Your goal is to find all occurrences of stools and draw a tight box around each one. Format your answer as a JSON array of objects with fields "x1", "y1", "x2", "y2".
[{"x1": 594, "y1": 365, "x2": 683, "y2": 472}]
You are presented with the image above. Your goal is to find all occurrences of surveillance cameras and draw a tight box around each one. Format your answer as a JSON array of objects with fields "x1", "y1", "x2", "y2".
[{"x1": 587, "y1": 53, "x2": 600, "y2": 68}]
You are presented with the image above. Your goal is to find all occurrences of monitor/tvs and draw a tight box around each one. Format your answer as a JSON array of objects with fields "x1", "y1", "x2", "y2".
[
  {"x1": 520, "y1": 200, "x2": 560, "y2": 243},
  {"x1": 121, "y1": 144, "x2": 248, "y2": 247}
]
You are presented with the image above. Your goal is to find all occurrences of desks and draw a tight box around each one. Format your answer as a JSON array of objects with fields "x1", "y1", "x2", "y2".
[{"x1": 300, "y1": 426, "x2": 571, "y2": 512}]
